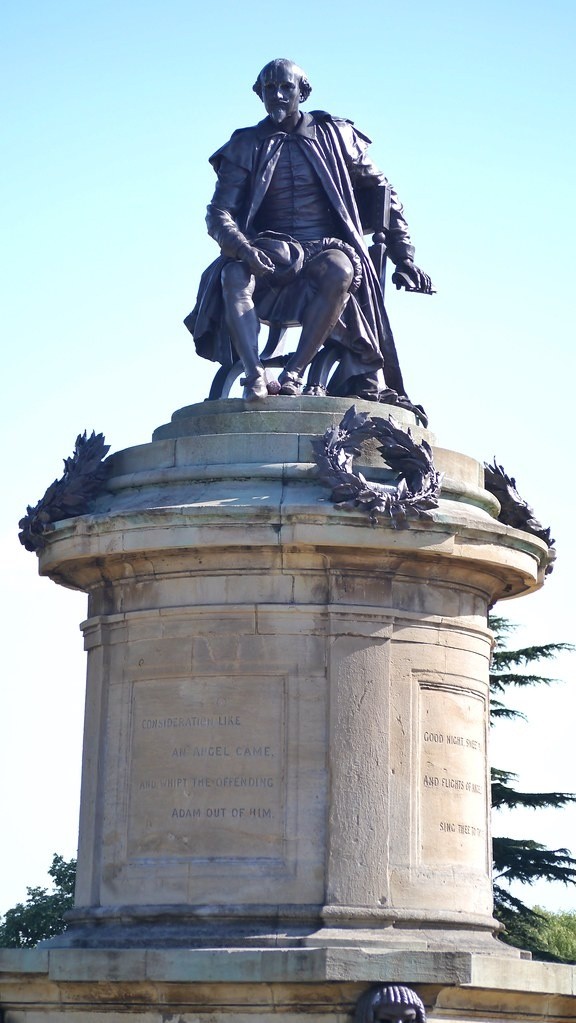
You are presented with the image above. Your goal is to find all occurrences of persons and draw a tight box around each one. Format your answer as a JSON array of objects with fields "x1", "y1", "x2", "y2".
[
  {"x1": 205, "y1": 57, "x2": 430, "y2": 401},
  {"x1": 355, "y1": 985, "x2": 427, "y2": 1023}
]
